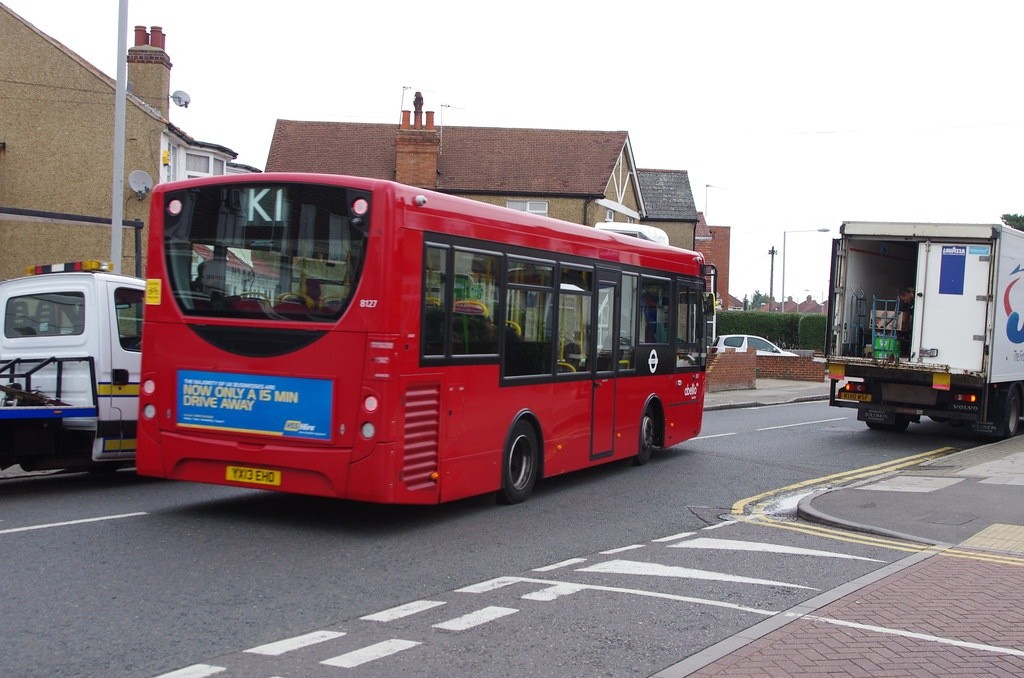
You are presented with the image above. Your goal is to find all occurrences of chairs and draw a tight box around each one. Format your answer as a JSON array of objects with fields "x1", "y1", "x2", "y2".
[
  {"x1": 13, "y1": 302, "x2": 28, "y2": 326},
  {"x1": 231, "y1": 292, "x2": 521, "y2": 342},
  {"x1": 609, "y1": 360, "x2": 630, "y2": 370},
  {"x1": 557, "y1": 363, "x2": 579, "y2": 372},
  {"x1": 36, "y1": 300, "x2": 57, "y2": 334}
]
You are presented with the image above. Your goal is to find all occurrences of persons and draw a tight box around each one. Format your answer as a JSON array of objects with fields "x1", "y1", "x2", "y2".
[
  {"x1": 900, "y1": 286, "x2": 918, "y2": 311},
  {"x1": 558, "y1": 343, "x2": 587, "y2": 372}
]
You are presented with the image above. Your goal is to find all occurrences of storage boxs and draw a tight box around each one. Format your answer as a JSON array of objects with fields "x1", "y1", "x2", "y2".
[{"x1": 869, "y1": 310, "x2": 912, "y2": 362}]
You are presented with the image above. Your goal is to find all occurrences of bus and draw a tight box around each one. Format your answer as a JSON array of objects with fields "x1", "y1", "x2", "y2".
[{"x1": 137, "y1": 172, "x2": 718, "y2": 505}]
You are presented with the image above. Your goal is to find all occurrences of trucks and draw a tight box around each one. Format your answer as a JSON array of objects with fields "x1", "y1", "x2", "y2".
[
  {"x1": 0, "y1": 260, "x2": 148, "y2": 476},
  {"x1": 824, "y1": 220, "x2": 1024, "y2": 438}
]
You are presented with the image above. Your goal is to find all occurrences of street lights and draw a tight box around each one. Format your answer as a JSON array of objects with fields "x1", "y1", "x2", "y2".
[
  {"x1": 797, "y1": 289, "x2": 810, "y2": 314},
  {"x1": 782, "y1": 229, "x2": 830, "y2": 313}
]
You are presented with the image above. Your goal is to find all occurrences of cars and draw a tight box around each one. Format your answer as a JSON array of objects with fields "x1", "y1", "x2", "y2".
[{"x1": 708, "y1": 334, "x2": 799, "y2": 356}]
